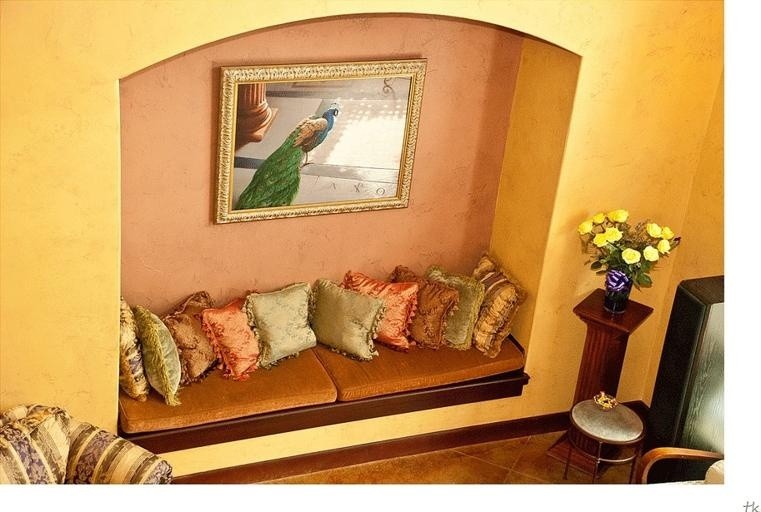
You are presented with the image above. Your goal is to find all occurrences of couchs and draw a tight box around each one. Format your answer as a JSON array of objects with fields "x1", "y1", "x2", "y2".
[{"x1": 0, "y1": 405, "x2": 171, "y2": 484}]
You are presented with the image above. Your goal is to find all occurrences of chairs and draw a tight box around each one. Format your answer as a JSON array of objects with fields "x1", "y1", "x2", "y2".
[{"x1": 636, "y1": 447, "x2": 724, "y2": 485}]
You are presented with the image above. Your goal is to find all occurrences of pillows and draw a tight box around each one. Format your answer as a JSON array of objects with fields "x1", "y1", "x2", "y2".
[
  {"x1": 200, "y1": 299, "x2": 262, "y2": 382},
  {"x1": 246, "y1": 283, "x2": 317, "y2": 369},
  {"x1": 120, "y1": 288, "x2": 219, "y2": 409},
  {"x1": 465, "y1": 251, "x2": 528, "y2": 359},
  {"x1": 309, "y1": 278, "x2": 387, "y2": 362},
  {"x1": 339, "y1": 271, "x2": 418, "y2": 354},
  {"x1": 423, "y1": 263, "x2": 484, "y2": 351},
  {"x1": 383, "y1": 264, "x2": 456, "y2": 351}
]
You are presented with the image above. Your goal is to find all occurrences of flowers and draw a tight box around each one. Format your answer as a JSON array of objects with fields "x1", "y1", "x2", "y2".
[{"x1": 577, "y1": 210, "x2": 679, "y2": 292}]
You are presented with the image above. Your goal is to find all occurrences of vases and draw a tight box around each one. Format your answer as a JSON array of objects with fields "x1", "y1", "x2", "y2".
[{"x1": 602, "y1": 264, "x2": 632, "y2": 317}]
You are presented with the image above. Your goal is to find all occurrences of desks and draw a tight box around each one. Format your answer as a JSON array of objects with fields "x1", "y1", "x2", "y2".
[{"x1": 547, "y1": 402, "x2": 644, "y2": 485}]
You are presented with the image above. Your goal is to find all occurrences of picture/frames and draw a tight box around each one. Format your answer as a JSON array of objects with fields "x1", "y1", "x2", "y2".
[{"x1": 211, "y1": 57, "x2": 428, "y2": 224}]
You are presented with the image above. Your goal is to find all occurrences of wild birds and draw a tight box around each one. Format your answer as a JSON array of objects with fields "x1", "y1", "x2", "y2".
[{"x1": 233, "y1": 108, "x2": 341, "y2": 211}]
